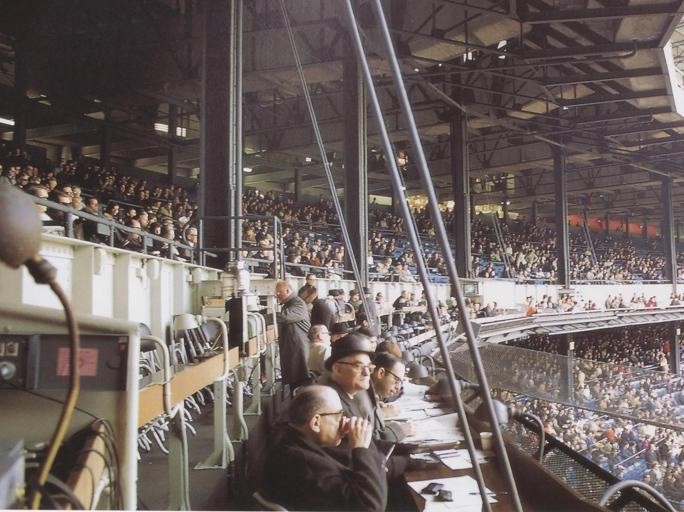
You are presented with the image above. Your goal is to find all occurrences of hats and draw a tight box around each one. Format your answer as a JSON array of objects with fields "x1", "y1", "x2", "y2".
[
  {"x1": 324, "y1": 334, "x2": 376, "y2": 371},
  {"x1": 327, "y1": 287, "x2": 372, "y2": 297}
]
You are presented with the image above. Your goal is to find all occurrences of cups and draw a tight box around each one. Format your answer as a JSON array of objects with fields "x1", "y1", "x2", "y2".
[
  {"x1": 341, "y1": 416, "x2": 352, "y2": 436},
  {"x1": 479, "y1": 431, "x2": 494, "y2": 451}
]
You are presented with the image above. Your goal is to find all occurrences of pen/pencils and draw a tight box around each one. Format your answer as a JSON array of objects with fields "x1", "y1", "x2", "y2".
[
  {"x1": 386, "y1": 444, "x2": 396, "y2": 463},
  {"x1": 469, "y1": 492, "x2": 508, "y2": 495}
]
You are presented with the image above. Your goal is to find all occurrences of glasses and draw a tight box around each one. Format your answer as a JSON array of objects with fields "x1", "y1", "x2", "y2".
[{"x1": 336, "y1": 360, "x2": 376, "y2": 375}]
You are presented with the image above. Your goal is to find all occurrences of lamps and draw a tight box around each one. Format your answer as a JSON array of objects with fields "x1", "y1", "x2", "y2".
[
  {"x1": 0, "y1": 184, "x2": 88, "y2": 510},
  {"x1": 136, "y1": 310, "x2": 266, "y2": 377},
  {"x1": 380, "y1": 318, "x2": 545, "y2": 463}
]
